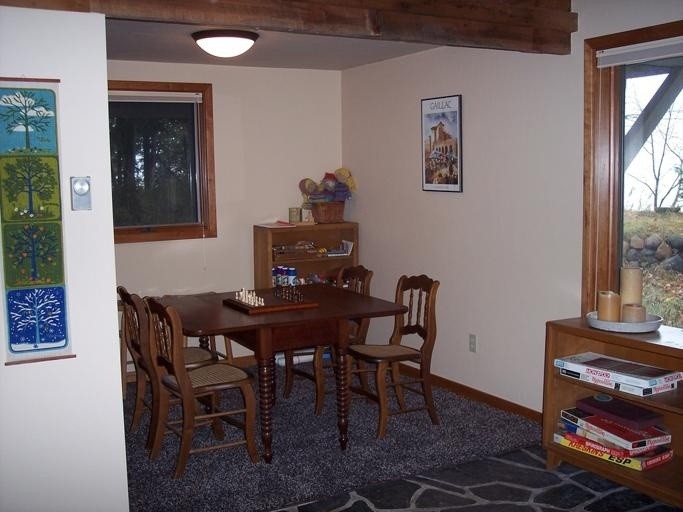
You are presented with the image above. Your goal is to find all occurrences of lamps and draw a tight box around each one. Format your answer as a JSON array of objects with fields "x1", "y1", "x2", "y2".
[{"x1": 189, "y1": 28, "x2": 260, "y2": 60}]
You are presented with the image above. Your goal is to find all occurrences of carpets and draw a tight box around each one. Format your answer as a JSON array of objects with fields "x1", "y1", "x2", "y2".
[{"x1": 119, "y1": 355, "x2": 542, "y2": 512}]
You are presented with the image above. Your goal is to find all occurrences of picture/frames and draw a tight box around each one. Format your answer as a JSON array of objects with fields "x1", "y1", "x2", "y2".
[{"x1": 420, "y1": 94, "x2": 464, "y2": 193}]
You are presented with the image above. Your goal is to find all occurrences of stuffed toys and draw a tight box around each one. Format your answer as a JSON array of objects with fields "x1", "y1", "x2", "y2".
[{"x1": 298, "y1": 168, "x2": 357, "y2": 202}]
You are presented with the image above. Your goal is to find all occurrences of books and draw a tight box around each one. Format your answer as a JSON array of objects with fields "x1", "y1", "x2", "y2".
[{"x1": 327, "y1": 240, "x2": 353, "y2": 257}]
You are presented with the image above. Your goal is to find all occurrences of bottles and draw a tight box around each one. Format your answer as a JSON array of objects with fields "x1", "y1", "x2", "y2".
[{"x1": 272, "y1": 265, "x2": 297, "y2": 288}]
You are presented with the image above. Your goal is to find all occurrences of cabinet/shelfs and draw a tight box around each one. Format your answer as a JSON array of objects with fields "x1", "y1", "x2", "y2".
[
  {"x1": 542, "y1": 317, "x2": 683, "y2": 510},
  {"x1": 253, "y1": 221, "x2": 358, "y2": 290}
]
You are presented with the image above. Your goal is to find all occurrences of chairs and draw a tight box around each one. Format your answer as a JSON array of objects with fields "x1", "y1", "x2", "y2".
[
  {"x1": 116, "y1": 283, "x2": 259, "y2": 481},
  {"x1": 282, "y1": 264, "x2": 440, "y2": 437}
]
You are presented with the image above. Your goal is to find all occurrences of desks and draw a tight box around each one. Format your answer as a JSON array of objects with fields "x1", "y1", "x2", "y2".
[{"x1": 157, "y1": 281, "x2": 409, "y2": 466}]
